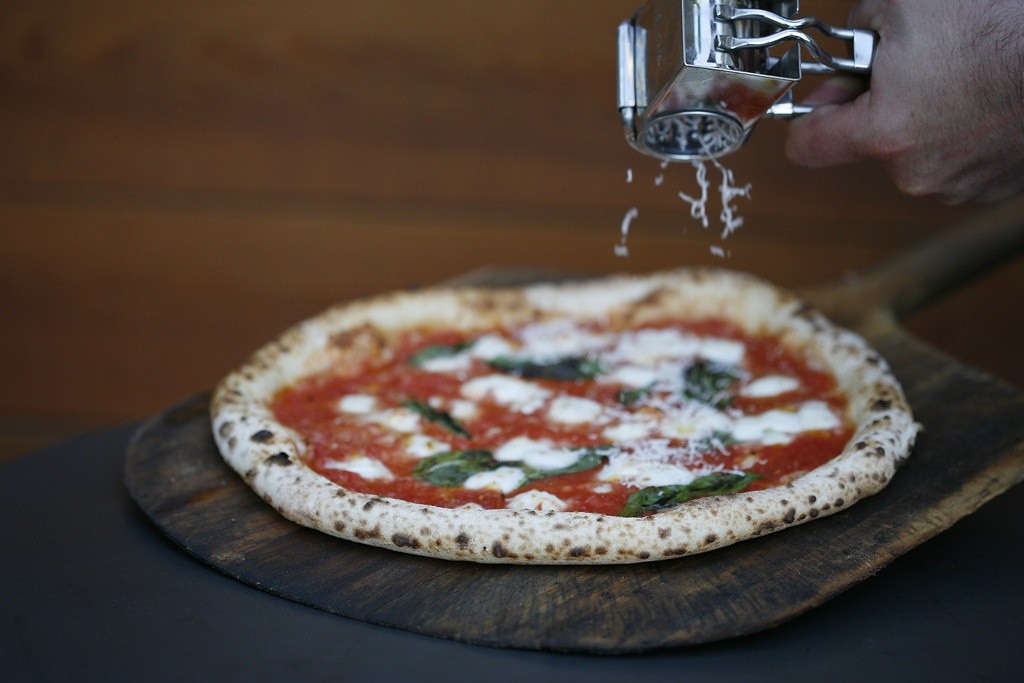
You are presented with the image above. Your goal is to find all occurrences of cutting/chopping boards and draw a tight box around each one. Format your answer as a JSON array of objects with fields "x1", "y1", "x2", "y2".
[{"x1": 123, "y1": 201, "x2": 1023, "y2": 655}]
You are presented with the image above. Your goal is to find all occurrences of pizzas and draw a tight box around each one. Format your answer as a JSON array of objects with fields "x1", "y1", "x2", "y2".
[{"x1": 208, "y1": 265, "x2": 917, "y2": 565}]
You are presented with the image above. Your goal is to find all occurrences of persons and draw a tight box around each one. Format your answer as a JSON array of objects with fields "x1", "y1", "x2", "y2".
[{"x1": 785, "y1": 0, "x2": 1024, "y2": 204}]
